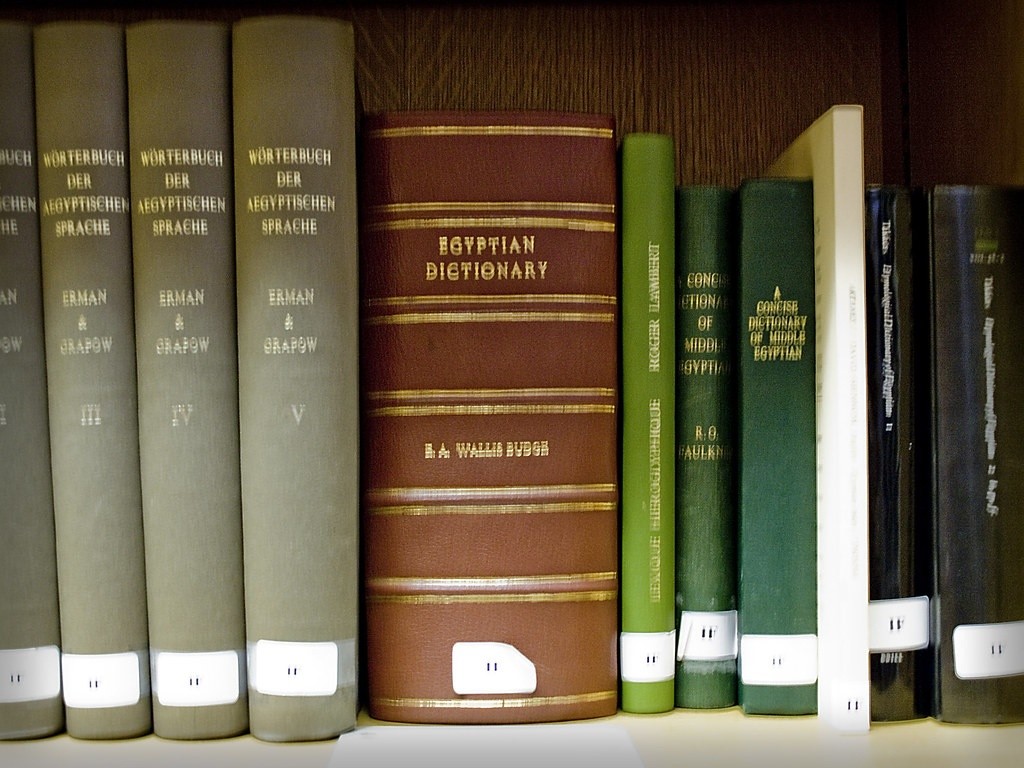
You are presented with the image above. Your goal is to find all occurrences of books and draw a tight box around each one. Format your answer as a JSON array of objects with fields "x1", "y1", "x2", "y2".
[
  {"x1": 0, "y1": 18, "x2": 365, "y2": 741},
  {"x1": 355, "y1": 110, "x2": 618, "y2": 725},
  {"x1": 864, "y1": 182, "x2": 1023, "y2": 723},
  {"x1": 621, "y1": 133, "x2": 815, "y2": 713}
]
